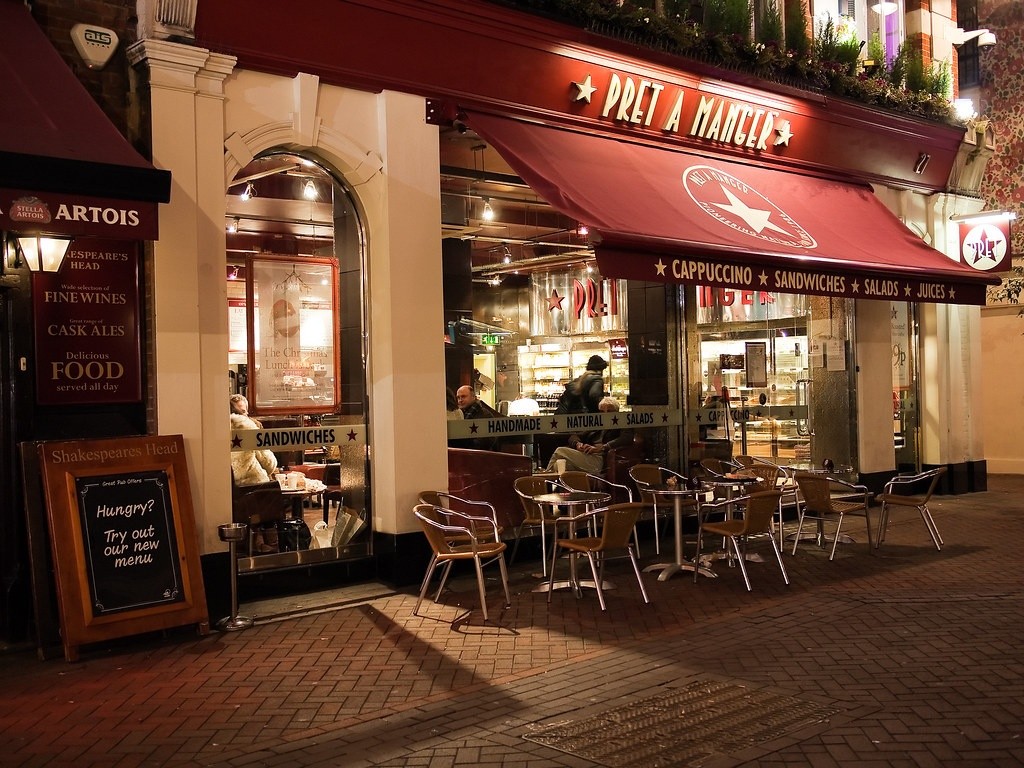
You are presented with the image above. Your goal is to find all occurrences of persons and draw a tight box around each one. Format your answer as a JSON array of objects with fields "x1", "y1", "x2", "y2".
[
  {"x1": 555, "y1": 355, "x2": 607, "y2": 440},
  {"x1": 545, "y1": 398, "x2": 633, "y2": 475},
  {"x1": 229, "y1": 395, "x2": 283, "y2": 553},
  {"x1": 446, "y1": 387, "x2": 465, "y2": 420},
  {"x1": 457, "y1": 385, "x2": 497, "y2": 451}
]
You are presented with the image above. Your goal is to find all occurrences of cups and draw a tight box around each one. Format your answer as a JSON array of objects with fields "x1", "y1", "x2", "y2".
[
  {"x1": 286, "y1": 474, "x2": 297, "y2": 488},
  {"x1": 275, "y1": 473, "x2": 286, "y2": 488}
]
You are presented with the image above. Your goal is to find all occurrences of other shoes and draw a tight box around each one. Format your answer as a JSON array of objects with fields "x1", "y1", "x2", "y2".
[{"x1": 255, "y1": 544, "x2": 272, "y2": 552}]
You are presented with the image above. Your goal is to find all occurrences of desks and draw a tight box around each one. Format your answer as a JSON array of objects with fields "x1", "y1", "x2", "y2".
[
  {"x1": 640, "y1": 482, "x2": 718, "y2": 582},
  {"x1": 532, "y1": 492, "x2": 612, "y2": 599},
  {"x1": 699, "y1": 476, "x2": 765, "y2": 568},
  {"x1": 281, "y1": 482, "x2": 325, "y2": 521}
]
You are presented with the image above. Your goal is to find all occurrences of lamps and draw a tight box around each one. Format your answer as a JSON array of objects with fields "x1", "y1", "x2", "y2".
[{"x1": 15, "y1": 235, "x2": 75, "y2": 273}]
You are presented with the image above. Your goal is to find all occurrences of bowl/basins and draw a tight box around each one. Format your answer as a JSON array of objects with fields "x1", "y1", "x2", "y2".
[{"x1": 218, "y1": 523, "x2": 247, "y2": 538}]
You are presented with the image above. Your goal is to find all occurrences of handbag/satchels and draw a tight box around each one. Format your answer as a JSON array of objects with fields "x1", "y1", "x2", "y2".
[
  {"x1": 278, "y1": 518, "x2": 311, "y2": 552},
  {"x1": 330, "y1": 507, "x2": 367, "y2": 547},
  {"x1": 309, "y1": 521, "x2": 333, "y2": 549},
  {"x1": 554, "y1": 389, "x2": 583, "y2": 416}
]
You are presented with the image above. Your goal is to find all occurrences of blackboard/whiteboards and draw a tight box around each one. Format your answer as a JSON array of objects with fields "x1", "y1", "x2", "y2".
[{"x1": 39, "y1": 434, "x2": 208, "y2": 644}]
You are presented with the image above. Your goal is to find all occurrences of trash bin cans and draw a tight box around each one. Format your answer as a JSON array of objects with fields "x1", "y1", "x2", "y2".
[{"x1": 698, "y1": 438, "x2": 734, "y2": 476}]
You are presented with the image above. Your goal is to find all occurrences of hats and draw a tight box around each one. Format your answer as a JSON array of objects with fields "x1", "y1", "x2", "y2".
[{"x1": 586, "y1": 355, "x2": 607, "y2": 371}]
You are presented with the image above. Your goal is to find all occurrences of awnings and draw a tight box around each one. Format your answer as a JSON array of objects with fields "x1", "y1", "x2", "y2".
[
  {"x1": 455, "y1": 108, "x2": 1003, "y2": 307},
  {"x1": 0, "y1": 0, "x2": 171, "y2": 240}
]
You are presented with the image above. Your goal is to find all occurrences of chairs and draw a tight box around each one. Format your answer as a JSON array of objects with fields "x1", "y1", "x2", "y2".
[
  {"x1": 510, "y1": 471, "x2": 649, "y2": 611},
  {"x1": 700, "y1": 453, "x2": 791, "y2": 591},
  {"x1": 875, "y1": 468, "x2": 949, "y2": 551},
  {"x1": 321, "y1": 465, "x2": 345, "y2": 527},
  {"x1": 628, "y1": 465, "x2": 704, "y2": 555},
  {"x1": 412, "y1": 491, "x2": 511, "y2": 621},
  {"x1": 790, "y1": 471, "x2": 874, "y2": 562},
  {"x1": 231, "y1": 465, "x2": 285, "y2": 557}
]
